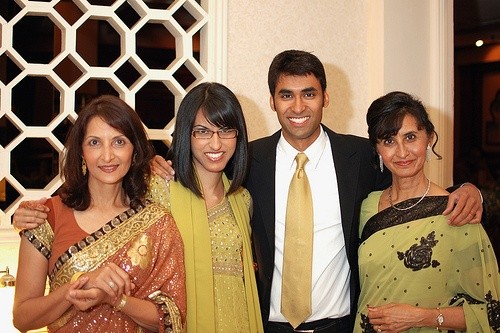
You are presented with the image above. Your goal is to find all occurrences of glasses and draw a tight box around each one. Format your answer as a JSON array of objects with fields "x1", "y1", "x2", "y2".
[{"x1": 191, "y1": 127, "x2": 238, "y2": 140}]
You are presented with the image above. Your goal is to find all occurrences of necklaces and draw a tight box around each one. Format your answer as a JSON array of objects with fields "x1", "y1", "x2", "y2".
[{"x1": 388, "y1": 178, "x2": 431, "y2": 211}]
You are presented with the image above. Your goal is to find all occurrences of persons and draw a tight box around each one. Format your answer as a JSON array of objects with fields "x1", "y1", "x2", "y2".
[
  {"x1": 148, "y1": 49, "x2": 486, "y2": 333},
  {"x1": 13, "y1": 80, "x2": 263, "y2": 333},
  {"x1": 344, "y1": 90, "x2": 500, "y2": 333},
  {"x1": 11, "y1": 97, "x2": 188, "y2": 333}
]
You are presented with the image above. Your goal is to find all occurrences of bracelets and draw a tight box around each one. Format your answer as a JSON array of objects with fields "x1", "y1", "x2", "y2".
[{"x1": 116, "y1": 294, "x2": 126, "y2": 311}]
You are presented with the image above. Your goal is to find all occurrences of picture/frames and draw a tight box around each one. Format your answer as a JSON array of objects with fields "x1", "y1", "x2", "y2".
[{"x1": 471, "y1": 62, "x2": 500, "y2": 158}]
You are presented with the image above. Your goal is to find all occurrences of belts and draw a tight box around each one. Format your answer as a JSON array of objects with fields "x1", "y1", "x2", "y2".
[{"x1": 267, "y1": 314, "x2": 352, "y2": 333}]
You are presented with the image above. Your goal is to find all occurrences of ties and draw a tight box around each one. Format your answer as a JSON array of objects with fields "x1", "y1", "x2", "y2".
[{"x1": 280, "y1": 152, "x2": 314, "y2": 330}]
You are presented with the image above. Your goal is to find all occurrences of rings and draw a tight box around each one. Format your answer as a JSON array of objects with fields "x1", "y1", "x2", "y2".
[
  {"x1": 108, "y1": 281, "x2": 115, "y2": 288},
  {"x1": 377, "y1": 324, "x2": 381, "y2": 333}
]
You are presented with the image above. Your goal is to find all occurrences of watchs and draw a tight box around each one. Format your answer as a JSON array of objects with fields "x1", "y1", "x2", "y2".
[{"x1": 436, "y1": 307, "x2": 444, "y2": 330}]
